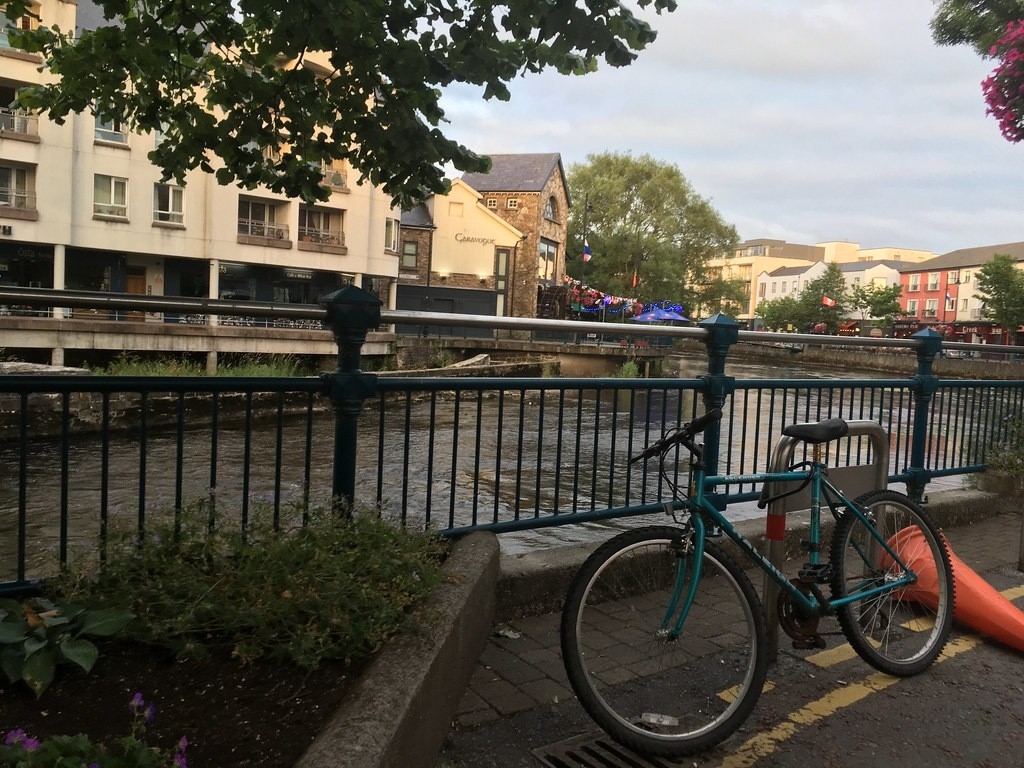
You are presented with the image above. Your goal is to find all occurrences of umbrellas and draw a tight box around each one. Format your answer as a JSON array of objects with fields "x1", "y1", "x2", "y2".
[
  {"x1": 654, "y1": 311, "x2": 690, "y2": 326},
  {"x1": 628, "y1": 309, "x2": 668, "y2": 324}
]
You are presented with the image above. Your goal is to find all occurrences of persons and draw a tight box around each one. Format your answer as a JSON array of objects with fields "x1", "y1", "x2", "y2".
[
  {"x1": 821, "y1": 321, "x2": 825, "y2": 334},
  {"x1": 813, "y1": 322, "x2": 819, "y2": 335}
]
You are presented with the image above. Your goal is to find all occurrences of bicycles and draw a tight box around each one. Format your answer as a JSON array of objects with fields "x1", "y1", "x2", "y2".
[{"x1": 559, "y1": 412, "x2": 958, "y2": 755}]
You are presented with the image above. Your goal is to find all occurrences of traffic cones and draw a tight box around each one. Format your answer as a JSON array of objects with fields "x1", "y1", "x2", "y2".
[{"x1": 877, "y1": 521, "x2": 1024, "y2": 654}]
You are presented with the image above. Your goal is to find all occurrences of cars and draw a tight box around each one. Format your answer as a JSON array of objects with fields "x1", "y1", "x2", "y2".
[{"x1": 941, "y1": 348, "x2": 966, "y2": 360}]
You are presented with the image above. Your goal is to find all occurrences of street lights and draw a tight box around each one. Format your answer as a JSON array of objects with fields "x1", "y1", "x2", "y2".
[{"x1": 575, "y1": 191, "x2": 598, "y2": 344}]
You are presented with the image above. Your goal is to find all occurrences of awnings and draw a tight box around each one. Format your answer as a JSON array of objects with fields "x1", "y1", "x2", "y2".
[
  {"x1": 837, "y1": 321, "x2": 857, "y2": 329},
  {"x1": 976, "y1": 301, "x2": 985, "y2": 310}
]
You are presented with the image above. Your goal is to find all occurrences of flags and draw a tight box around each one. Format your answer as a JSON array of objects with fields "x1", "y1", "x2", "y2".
[
  {"x1": 946, "y1": 291, "x2": 950, "y2": 301},
  {"x1": 824, "y1": 297, "x2": 835, "y2": 306}
]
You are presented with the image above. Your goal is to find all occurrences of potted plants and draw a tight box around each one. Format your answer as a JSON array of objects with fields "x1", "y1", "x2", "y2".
[
  {"x1": 641, "y1": 337, "x2": 649, "y2": 345},
  {"x1": 303, "y1": 232, "x2": 312, "y2": 241},
  {"x1": 633, "y1": 337, "x2": 642, "y2": 347},
  {"x1": 620, "y1": 338, "x2": 628, "y2": 346}
]
